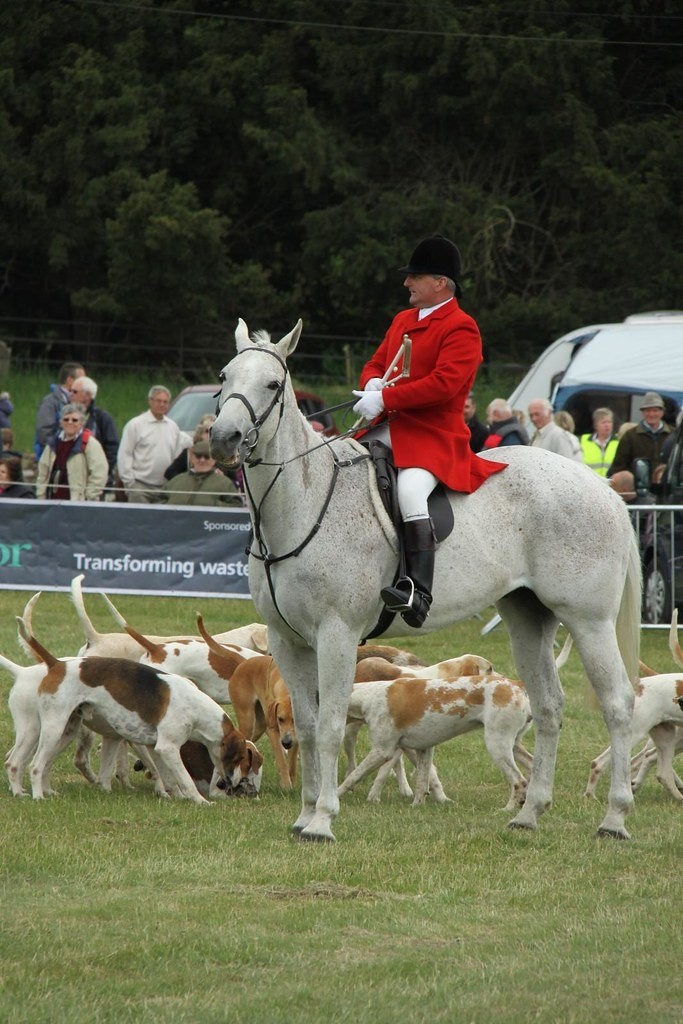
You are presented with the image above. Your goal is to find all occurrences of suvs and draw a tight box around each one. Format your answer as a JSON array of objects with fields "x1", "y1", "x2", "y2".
[{"x1": 632, "y1": 408, "x2": 682, "y2": 625}]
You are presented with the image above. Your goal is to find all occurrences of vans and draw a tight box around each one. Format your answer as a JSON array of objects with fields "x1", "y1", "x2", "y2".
[{"x1": 507, "y1": 311, "x2": 683, "y2": 456}]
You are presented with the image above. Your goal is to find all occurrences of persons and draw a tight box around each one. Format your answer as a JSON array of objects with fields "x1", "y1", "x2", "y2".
[
  {"x1": 352, "y1": 237, "x2": 482, "y2": 627},
  {"x1": 461, "y1": 389, "x2": 683, "y2": 534},
  {"x1": 1, "y1": 363, "x2": 324, "y2": 508}
]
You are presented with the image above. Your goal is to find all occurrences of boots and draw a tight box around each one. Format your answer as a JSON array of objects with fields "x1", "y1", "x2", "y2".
[{"x1": 381, "y1": 517, "x2": 437, "y2": 628}]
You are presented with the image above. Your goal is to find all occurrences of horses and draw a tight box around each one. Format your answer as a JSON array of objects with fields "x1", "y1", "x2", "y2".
[{"x1": 207, "y1": 317, "x2": 643, "y2": 841}]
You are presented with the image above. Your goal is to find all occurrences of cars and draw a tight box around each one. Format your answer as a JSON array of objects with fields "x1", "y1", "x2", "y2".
[{"x1": 113, "y1": 383, "x2": 343, "y2": 504}]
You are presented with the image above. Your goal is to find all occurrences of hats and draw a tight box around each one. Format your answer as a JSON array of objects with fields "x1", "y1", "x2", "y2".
[
  {"x1": 398, "y1": 232, "x2": 462, "y2": 300},
  {"x1": 192, "y1": 441, "x2": 210, "y2": 457},
  {"x1": 608, "y1": 471, "x2": 637, "y2": 501},
  {"x1": 639, "y1": 392, "x2": 664, "y2": 410}
]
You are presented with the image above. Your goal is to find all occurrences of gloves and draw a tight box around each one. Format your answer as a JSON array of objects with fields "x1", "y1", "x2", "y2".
[{"x1": 352, "y1": 378, "x2": 388, "y2": 420}]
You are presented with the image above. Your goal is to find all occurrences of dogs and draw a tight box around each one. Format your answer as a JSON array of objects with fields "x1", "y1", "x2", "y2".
[{"x1": 0, "y1": 574, "x2": 683, "y2": 810}]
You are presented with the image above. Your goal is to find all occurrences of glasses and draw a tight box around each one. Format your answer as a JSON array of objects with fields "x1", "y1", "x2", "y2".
[
  {"x1": 196, "y1": 456, "x2": 210, "y2": 460},
  {"x1": 63, "y1": 417, "x2": 79, "y2": 422},
  {"x1": 206, "y1": 429, "x2": 208, "y2": 433},
  {"x1": 69, "y1": 389, "x2": 80, "y2": 394}
]
